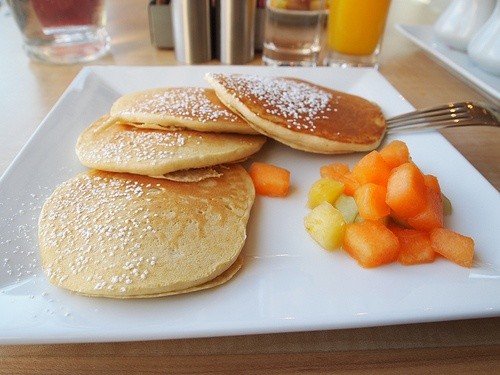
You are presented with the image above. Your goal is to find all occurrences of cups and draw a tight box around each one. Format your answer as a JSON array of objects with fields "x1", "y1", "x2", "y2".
[{"x1": 8, "y1": 1, "x2": 391, "y2": 67}]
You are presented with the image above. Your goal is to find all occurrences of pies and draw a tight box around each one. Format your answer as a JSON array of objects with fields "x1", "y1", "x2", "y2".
[{"x1": 36, "y1": 73, "x2": 387, "y2": 300}]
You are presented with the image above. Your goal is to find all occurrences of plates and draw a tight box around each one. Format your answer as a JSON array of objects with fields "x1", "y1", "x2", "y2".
[{"x1": 0, "y1": 57, "x2": 500, "y2": 333}]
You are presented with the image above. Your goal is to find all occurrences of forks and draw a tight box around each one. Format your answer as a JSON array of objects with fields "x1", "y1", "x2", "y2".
[{"x1": 384, "y1": 99, "x2": 500, "y2": 136}]
